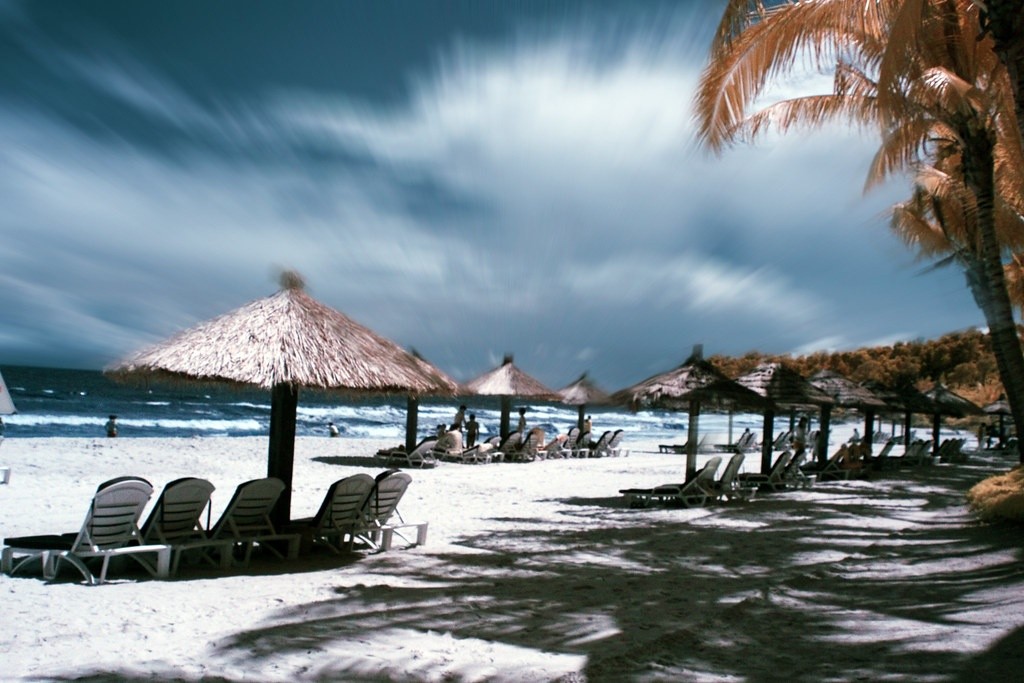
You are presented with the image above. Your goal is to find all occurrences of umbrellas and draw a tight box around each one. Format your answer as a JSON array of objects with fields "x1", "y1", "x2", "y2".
[
  {"x1": 406, "y1": 352, "x2": 470, "y2": 452},
  {"x1": 732, "y1": 359, "x2": 1013, "y2": 475},
  {"x1": 609, "y1": 344, "x2": 767, "y2": 478},
  {"x1": 462, "y1": 355, "x2": 565, "y2": 441},
  {"x1": 104, "y1": 273, "x2": 466, "y2": 532},
  {"x1": 557, "y1": 375, "x2": 612, "y2": 438}
]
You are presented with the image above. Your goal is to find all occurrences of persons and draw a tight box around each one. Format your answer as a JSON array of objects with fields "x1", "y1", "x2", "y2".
[
  {"x1": 793, "y1": 417, "x2": 808, "y2": 453},
  {"x1": 434, "y1": 424, "x2": 463, "y2": 456},
  {"x1": 739, "y1": 428, "x2": 750, "y2": 442},
  {"x1": 390, "y1": 445, "x2": 404, "y2": 452},
  {"x1": 453, "y1": 404, "x2": 467, "y2": 431},
  {"x1": 435, "y1": 423, "x2": 448, "y2": 438},
  {"x1": 851, "y1": 428, "x2": 860, "y2": 439},
  {"x1": 465, "y1": 414, "x2": 480, "y2": 449},
  {"x1": 585, "y1": 416, "x2": 592, "y2": 433},
  {"x1": 328, "y1": 423, "x2": 340, "y2": 436},
  {"x1": 976, "y1": 422, "x2": 986, "y2": 448},
  {"x1": 809, "y1": 431, "x2": 821, "y2": 462},
  {"x1": 104, "y1": 415, "x2": 118, "y2": 437},
  {"x1": 518, "y1": 408, "x2": 527, "y2": 433}
]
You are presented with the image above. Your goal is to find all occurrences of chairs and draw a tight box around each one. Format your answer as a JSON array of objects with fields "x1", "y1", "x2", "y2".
[
  {"x1": 618, "y1": 428, "x2": 967, "y2": 508},
  {"x1": 374, "y1": 428, "x2": 625, "y2": 468},
  {"x1": 0, "y1": 470, "x2": 429, "y2": 585}
]
